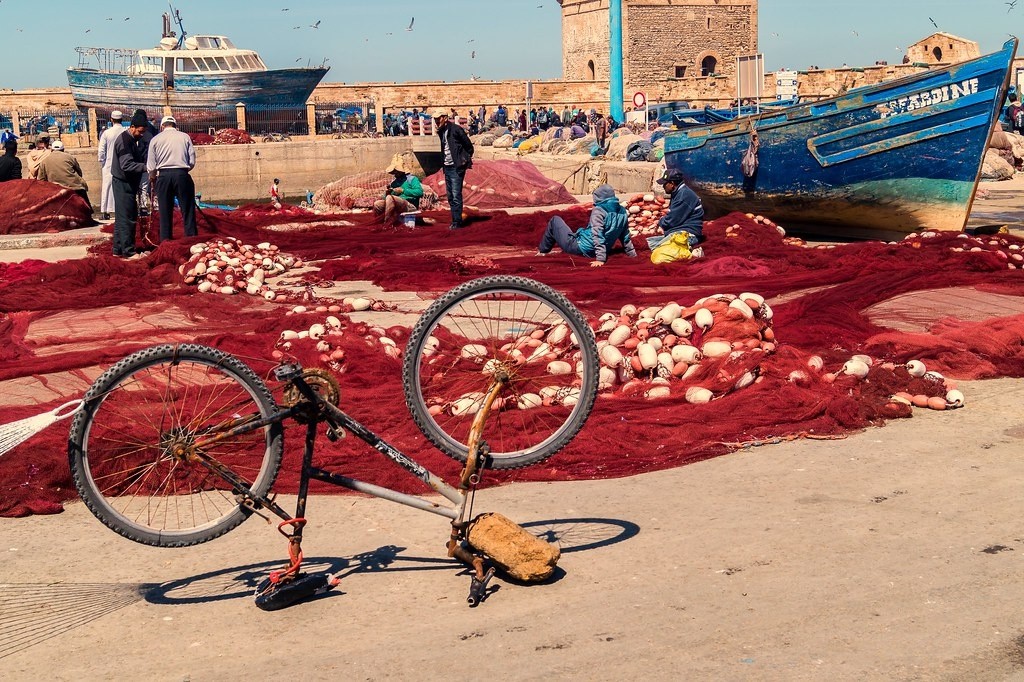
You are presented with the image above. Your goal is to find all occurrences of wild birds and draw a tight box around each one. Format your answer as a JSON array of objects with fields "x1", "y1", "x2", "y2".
[
  {"x1": 362, "y1": 38, "x2": 369, "y2": 42},
  {"x1": 16, "y1": 28, "x2": 24, "y2": 32},
  {"x1": 318, "y1": 56, "x2": 329, "y2": 67},
  {"x1": 403, "y1": 15, "x2": 415, "y2": 31},
  {"x1": 124, "y1": 17, "x2": 129, "y2": 21},
  {"x1": 772, "y1": 32, "x2": 779, "y2": 38},
  {"x1": 85, "y1": 30, "x2": 91, "y2": 33},
  {"x1": 471, "y1": 51, "x2": 477, "y2": 58},
  {"x1": 281, "y1": 8, "x2": 289, "y2": 11},
  {"x1": 293, "y1": 26, "x2": 300, "y2": 29},
  {"x1": 1004, "y1": 0, "x2": 1018, "y2": 14},
  {"x1": 470, "y1": 74, "x2": 480, "y2": 81},
  {"x1": 896, "y1": 45, "x2": 903, "y2": 52},
  {"x1": 929, "y1": 17, "x2": 938, "y2": 29},
  {"x1": 295, "y1": 57, "x2": 302, "y2": 61},
  {"x1": 309, "y1": 21, "x2": 320, "y2": 29},
  {"x1": 852, "y1": 31, "x2": 859, "y2": 37},
  {"x1": 106, "y1": 18, "x2": 113, "y2": 21},
  {"x1": 537, "y1": 5, "x2": 543, "y2": 8},
  {"x1": 468, "y1": 39, "x2": 474, "y2": 43},
  {"x1": 385, "y1": 33, "x2": 394, "y2": 36}
]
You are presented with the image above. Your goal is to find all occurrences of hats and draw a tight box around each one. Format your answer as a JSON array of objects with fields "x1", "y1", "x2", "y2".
[
  {"x1": 52, "y1": 141, "x2": 64, "y2": 150},
  {"x1": 160, "y1": 115, "x2": 176, "y2": 130},
  {"x1": 386, "y1": 154, "x2": 409, "y2": 174},
  {"x1": 432, "y1": 110, "x2": 447, "y2": 118},
  {"x1": 111, "y1": 110, "x2": 123, "y2": 119},
  {"x1": 656, "y1": 168, "x2": 683, "y2": 184},
  {"x1": 594, "y1": 109, "x2": 604, "y2": 114}
]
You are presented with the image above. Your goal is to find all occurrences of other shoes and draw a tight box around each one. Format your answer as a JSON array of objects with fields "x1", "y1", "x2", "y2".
[
  {"x1": 123, "y1": 251, "x2": 136, "y2": 257},
  {"x1": 449, "y1": 224, "x2": 457, "y2": 230},
  {"x1": 113, "y1": 250, "x2": 122, "y2": 256},
  {"x1": 99, "y1": 213, "x2": 109, "y2": 220},
  {"x1": 692, "y1": 246, "x2": 705, "y2": 258}
]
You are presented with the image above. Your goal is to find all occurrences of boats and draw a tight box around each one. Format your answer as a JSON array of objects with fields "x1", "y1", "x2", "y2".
[
  {"x1": 66, "y1": 1, "x2": 331, "y2": 130},
  {"x1": 663, "y1": 37, "x2": 1020, "y2": 243}
]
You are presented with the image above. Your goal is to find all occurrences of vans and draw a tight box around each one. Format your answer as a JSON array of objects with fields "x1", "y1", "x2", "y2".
[{"x1": 634, "y1": 101, "x2": 690, "y2": 124}]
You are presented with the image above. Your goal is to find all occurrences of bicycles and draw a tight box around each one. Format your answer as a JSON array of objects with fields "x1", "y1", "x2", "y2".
[{"x1": 66, "y1": 274, "x2": 601, "y2": 612}]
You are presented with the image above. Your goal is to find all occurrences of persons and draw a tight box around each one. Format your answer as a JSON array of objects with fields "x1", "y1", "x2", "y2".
[
  {"x1": 693, "y1": 97, "x2": 756, "y2": 109},
  {"x1": 449, "y1": 104, "x2": 516, "y2": 140},
  {"x1": 147, "y1": 115, "x2": 197, "y2": 239},
  {"x1": 374, "y1": 158, "x2": 423, "y2": 223},
  {"x1": 514, "y1": 104, "x2": 619, "y2": 148},
  {"x1": 315, "y1": 108, "x2": 419, "y2": 136},
  {"x1": 96, "y1": 109, "x2": 158, "y2": 256},
  {"x1": 272, "y1": 179, "x2": 283, "y2": 208},
  {"x1": 431, "y1": 108, "x2": 474, "y2": 230},
  {"x1": 780, "y1": 61, "x2": 887, "y2": 71},
  {"x1": 624, "y1": 107, "x2": 631, "y2": 119},
  {"x1": 535, "y1": 184, "x2": 637, "y2": 266},
  {"x1": 0, "y1": 122, "x2": 94, "y2": 214},
  {"x1": 649, "y1": 167, "x2": 704, "y2": 262},
  {"x1": 1005, "y1": 93, "x2": 1024, "y2": 171}
]
